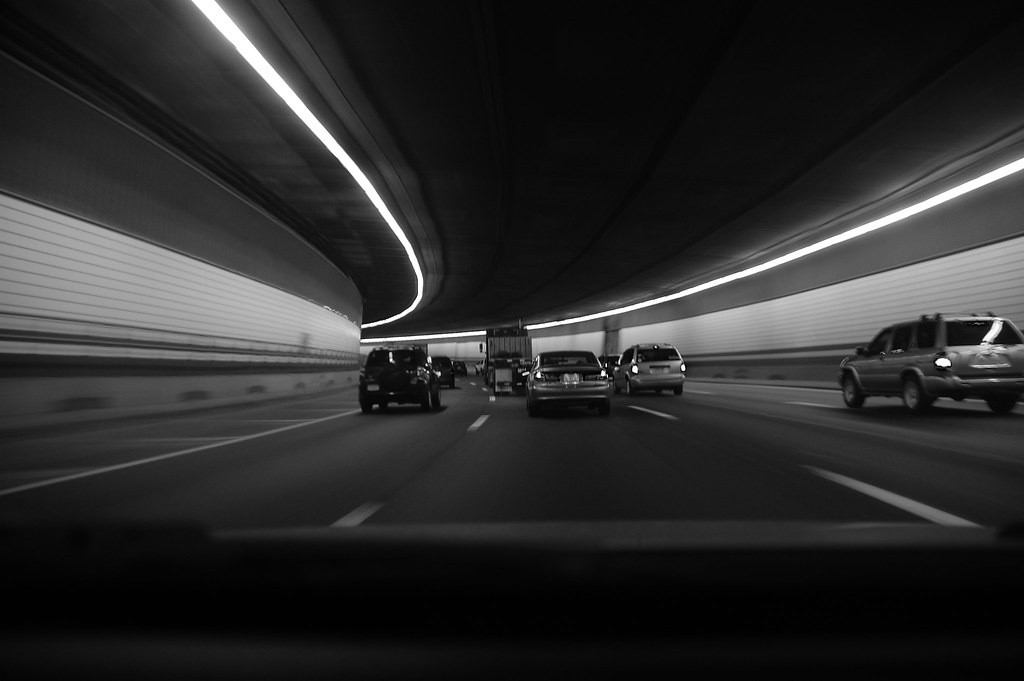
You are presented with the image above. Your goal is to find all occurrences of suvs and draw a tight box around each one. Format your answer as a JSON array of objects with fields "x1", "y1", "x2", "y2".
[
  {"x1": 837, "y1": 310, "x2": 1024, "y2": 415},
  {"x1": 358, "y1": 346, "x2": 441, "y2": 413}
]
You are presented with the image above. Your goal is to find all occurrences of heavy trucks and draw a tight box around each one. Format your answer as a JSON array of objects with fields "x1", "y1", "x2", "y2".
[{"x1": 479, "y1": 327, "x2": 534, "y2": 397}]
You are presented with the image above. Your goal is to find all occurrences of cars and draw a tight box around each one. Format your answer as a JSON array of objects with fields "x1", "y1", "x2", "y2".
[
  {"x1": 453, "y1": 361, "x2": 467, "y2": 377},
  {"x1": 427, "y1": 356, "x2": 456, "y2": 388},
  {"x1": 522, "y1": 351, "x2": 613, "y2": 417},
  {"x1": 597, "y1": 354, "x2": 622, "y2": 382},
  {"x1": 612, "y1": 343, "x2": 686, "y2": 396},
  {"x1": 475, "y1": 360, "x2": 484, "y2": 376}
]
